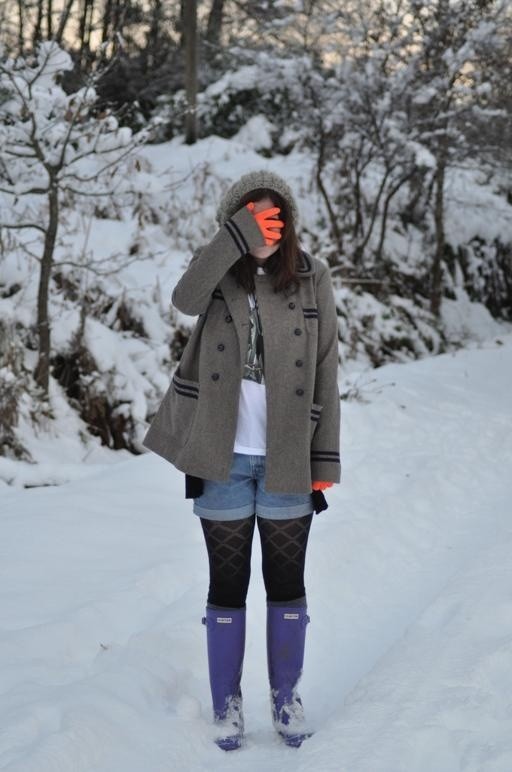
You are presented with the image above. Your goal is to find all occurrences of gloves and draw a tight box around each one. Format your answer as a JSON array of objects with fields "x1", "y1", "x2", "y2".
[
  {"x1": 247, "y1": 202, "x2": 284, "y2": 246},
  {"x1": 312, "y1": 481, "x2": 334, "y2": 491}
]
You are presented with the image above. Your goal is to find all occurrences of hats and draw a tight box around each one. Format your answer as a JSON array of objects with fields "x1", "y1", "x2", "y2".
[{"x1": 216, "y1": 171, "x2": 298, "y2": 229}]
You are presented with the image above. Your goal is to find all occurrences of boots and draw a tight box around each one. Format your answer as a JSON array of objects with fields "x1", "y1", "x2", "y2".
[
  {"x1": 202, "y1": 607, "x2": 244, "y2": 751},
  {"x1": 266, "y1": 607, "x2": 312, "y2": 748}
]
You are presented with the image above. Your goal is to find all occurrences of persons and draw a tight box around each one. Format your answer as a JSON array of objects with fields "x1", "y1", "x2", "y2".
[{"x1": 142, "y1": 170, "x2": 341, "y2": 749}]
